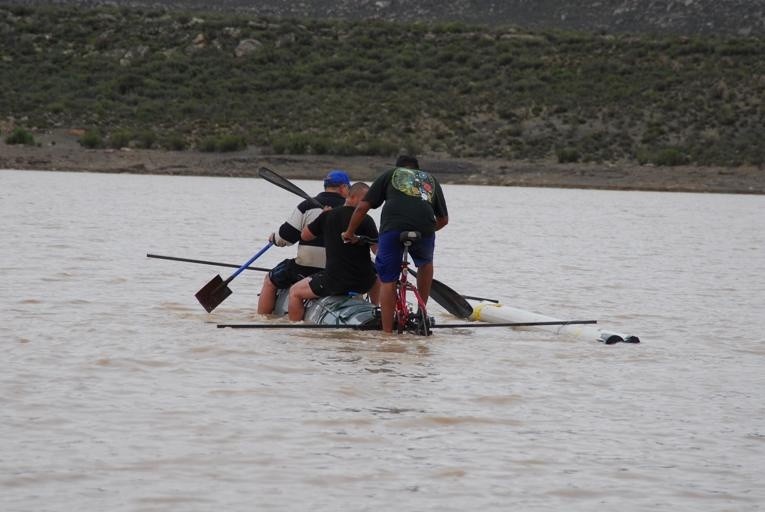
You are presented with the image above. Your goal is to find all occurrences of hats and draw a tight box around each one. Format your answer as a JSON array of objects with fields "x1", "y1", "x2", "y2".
[
  {"x1": 397, "y1": 156, "x2": 418, "y2": 167},
  {"x1": 324, "y1": 172, "x2": 351, "y2": 188}
]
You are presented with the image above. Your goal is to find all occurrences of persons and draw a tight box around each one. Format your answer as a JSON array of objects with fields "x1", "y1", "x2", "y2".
[
  {"x1": 255, "y1": 169, "x2": 351, "y2": 316},
  {"x1": 340, "y1": 153, "x2": 449, "y2": 334},
  {"x1": 288, "y1": 181, "x2": 380, "y2": 320}
]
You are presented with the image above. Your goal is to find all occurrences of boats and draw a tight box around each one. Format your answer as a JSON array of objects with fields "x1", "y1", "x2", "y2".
[{"x1": 270, "y1": 276, "x2": 641, "y2": 345}]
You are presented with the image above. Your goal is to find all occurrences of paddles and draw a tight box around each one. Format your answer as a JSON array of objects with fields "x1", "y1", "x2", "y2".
[
  {"x1": 256, "y1": 164, "x2": 475, "y2": 320},
  {"x1": 193, "y1": 244, "x2": 273, "y2": 316}
]
